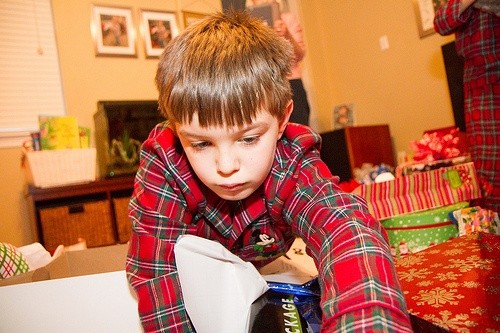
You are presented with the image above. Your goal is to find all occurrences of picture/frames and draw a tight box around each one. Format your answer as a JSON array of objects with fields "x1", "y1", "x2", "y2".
[
  {"x1": 412, "y1": 0, "x2": 437, "y2": 39},
  {"x1": 139, "y1": 9, "x2": 181, "y2": 58},
  {"x1": 91, "y1": 4, "x2": 138, "y2": 57},
  {"x1": 182, "y1": 10, "x2": 211, "y2": 30}
]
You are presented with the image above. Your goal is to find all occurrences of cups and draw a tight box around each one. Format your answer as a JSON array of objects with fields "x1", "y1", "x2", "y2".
[{"x1": 370, "y1": 168, "x2": 394, "y2": 184}]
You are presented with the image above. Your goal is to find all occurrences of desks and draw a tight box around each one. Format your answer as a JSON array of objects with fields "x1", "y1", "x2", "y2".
[{"x1": 320, "y1": 125, "x2": 396, "y2": 192}]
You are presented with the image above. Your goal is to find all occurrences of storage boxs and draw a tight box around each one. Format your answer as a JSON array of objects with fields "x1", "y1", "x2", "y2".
[
  {"x1": 24, "y1": 148, "x2": 97, "y2": 189},
  {"x1": 0, "y1": 154, "x2": 500, "y2": 333},
  {"x1": 94, "y1": 100, "x2": 167, "y2": 178}
]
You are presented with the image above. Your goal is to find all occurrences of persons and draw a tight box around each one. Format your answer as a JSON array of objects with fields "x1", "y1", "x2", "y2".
[
  {"x1": 125, "y1": 9, "x2": 415, "y2": 333},
  {"x1": 434, "y1": 0, "x2": 500, "y2": 216},
  {"x1": 252, "y1": 0, "x2": 310, "y2": 126}
]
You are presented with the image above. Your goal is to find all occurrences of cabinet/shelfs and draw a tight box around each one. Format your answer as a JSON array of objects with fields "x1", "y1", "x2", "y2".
[{"x1": 23, "y1": 175, "x2": 138, "y2": 252}]
